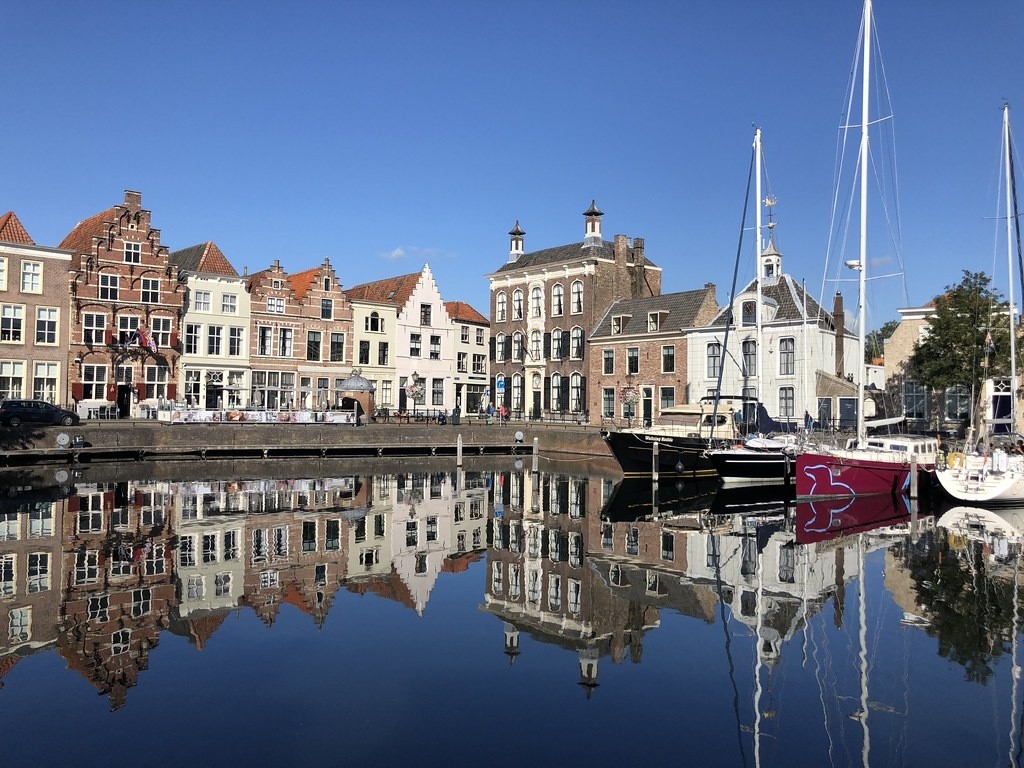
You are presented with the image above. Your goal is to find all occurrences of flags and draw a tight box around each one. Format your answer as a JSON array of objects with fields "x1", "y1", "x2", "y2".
[{"x1": 132, "y1": 328, "x2": 158, "y2": 354}]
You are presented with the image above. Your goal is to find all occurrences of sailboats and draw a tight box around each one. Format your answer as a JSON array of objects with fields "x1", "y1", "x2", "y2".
[{"x1": 598, "y1": 0, "x2": 1024, "y2": 507}]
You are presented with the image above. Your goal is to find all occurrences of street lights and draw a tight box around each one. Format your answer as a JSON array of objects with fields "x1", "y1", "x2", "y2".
[
  {"x1": 226, "y1": 372, "x2": 237, "y2": 409},
  {"x1": 411, "y1": 370, "x2": 420, "y2": 415},
  {"x1": 622, "y1": 367, "x2": 636, "y2": 428}
]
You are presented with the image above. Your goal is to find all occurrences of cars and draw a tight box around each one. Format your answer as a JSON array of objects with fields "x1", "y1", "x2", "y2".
[{"x1": 0, "y1": 399, "x2": 81, "y2": 426}]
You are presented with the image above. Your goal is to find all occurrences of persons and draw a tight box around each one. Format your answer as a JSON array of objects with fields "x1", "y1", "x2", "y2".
[
  {"x1": 735, "y1": 410, "x2": 743, "y2": 435},
  {"x1": 804, "y1": 411, "x2": 813, "y2": 429},
  {"x1": 438, "y1": 402, "x2": 509, "y2": 426},
  {"x1": 938, "y1": 438, "x2": 949, "y2": 470}
]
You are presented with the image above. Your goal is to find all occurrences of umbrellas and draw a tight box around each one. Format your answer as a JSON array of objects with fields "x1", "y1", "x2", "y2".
[{"x1": 218, "y1": 385, "x2": 332, "y2": 392}]
